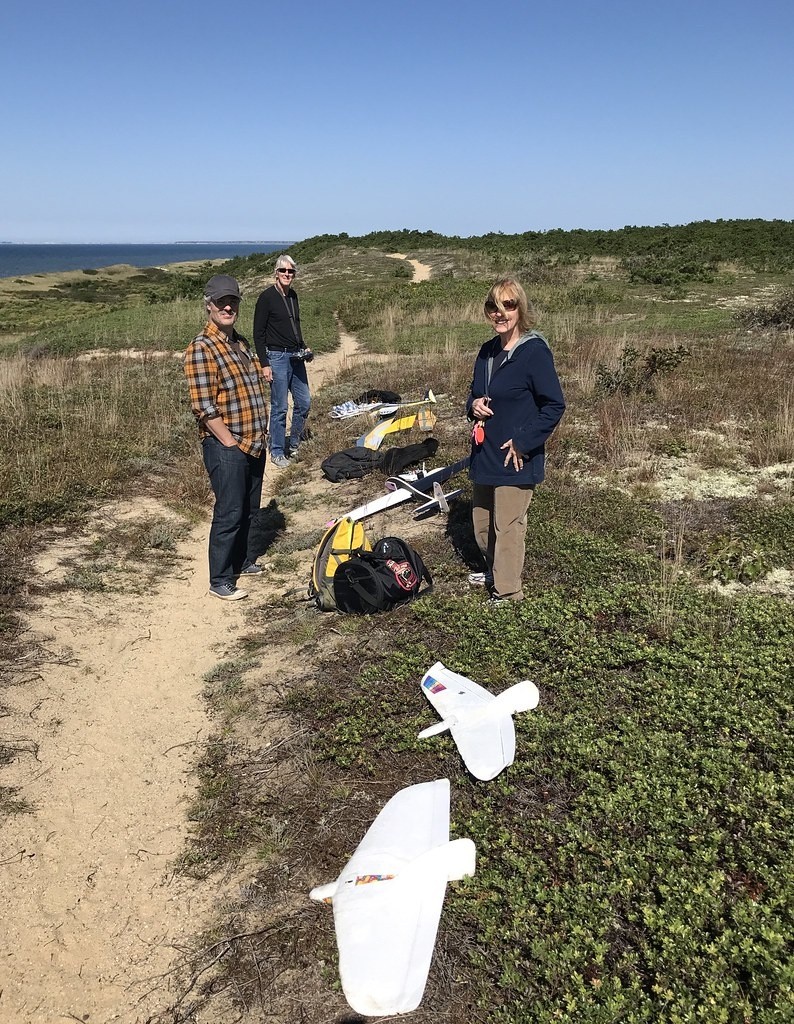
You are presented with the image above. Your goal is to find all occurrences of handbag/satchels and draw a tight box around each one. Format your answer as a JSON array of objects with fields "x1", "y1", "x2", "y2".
[{"x1": 334, "y1": 537, "x2": 431, "y2": 614}]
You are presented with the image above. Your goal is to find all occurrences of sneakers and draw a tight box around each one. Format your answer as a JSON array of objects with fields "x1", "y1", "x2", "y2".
[
  {"x1": 271, "y1": 456, "x2": 290, "y2": 468},
  {"x1": 235, "y1": 561, "x2": 275, "y2": 577},
  {"x1": 209, "y1": 584, "x2": 248, "y2": 601},
  {"x1": 290, "y1": 452, "x2": 298, "y2": 456},
  {"x1": 470, "y1": 571, "x2": 493, "y2": 585},
  {"x1": 482, "y1": 598, "x2": 505, "y2": 607}
]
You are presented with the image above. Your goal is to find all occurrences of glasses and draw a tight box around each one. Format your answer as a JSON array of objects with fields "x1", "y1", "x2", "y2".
[
  {"x1": 277, "y1": 268, "x2": 295, "y2": 274},
  {"x1": 485, "y1": 300, "x2": 517, "y2": 312}
]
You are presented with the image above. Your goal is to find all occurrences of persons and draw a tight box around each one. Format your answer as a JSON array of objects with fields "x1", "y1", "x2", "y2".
[
  {"x1": 466, "y1": 279, "x2": 565, "y2": 609},
  {"x1": 253, "y1": 255, "x2": 312, "y2": 468},
  {"x1": 183, "y1": 277, "x2": 271, "y2": 603}
]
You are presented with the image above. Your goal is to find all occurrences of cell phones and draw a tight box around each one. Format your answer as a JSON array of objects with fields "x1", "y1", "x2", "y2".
[{"x1": 483, "y1": 395, "x2": 489, "y2": 409}]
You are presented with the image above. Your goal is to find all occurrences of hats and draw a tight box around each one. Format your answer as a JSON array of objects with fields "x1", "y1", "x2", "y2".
[{"x1": 204, "y1": 275, "x2": 242, "y2": 301}]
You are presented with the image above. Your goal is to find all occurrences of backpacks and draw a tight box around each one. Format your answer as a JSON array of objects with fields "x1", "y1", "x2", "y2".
[
  {"x1": 309, "y1": 516, "x2": 372, "y2": 610},
  {"x1": 322, "y1": 446, "x2": 383, "y2": 480}
]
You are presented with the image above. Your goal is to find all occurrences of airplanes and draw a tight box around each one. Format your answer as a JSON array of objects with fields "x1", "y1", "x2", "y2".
[
  {"x1": 379, "y1": 389, "x2": 437, "y2": 418},
  {"x1": 324, "y1": 456, "x2": 471, "y2": 534}
]
